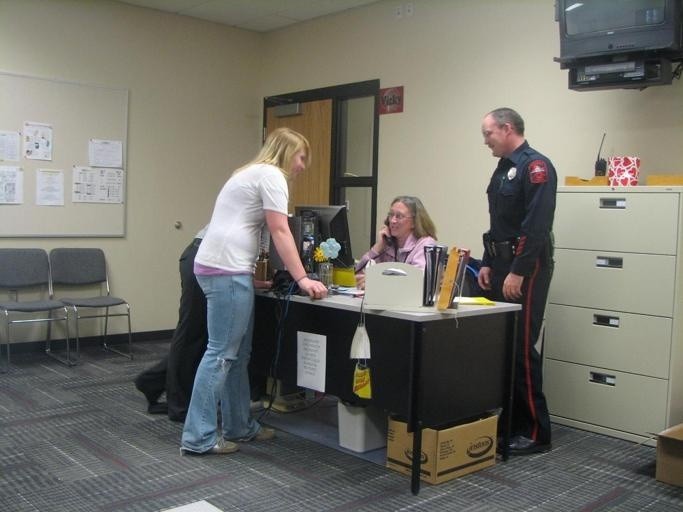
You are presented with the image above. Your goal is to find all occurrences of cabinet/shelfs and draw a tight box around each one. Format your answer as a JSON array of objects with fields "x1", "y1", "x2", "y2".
[{"x1": 541, "y1": 184, "x2": 682, "y2": 449}]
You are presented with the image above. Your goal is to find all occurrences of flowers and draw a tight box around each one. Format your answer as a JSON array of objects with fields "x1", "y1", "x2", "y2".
[{"x1": 313, "y1": 237, "x2": 342, "y2": 263}]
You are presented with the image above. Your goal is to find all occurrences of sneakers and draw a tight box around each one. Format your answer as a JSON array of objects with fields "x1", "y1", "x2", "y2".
[
  {"x1": 256, "y1": 426, "x2": 275, "y2": 441},
  {"x1": 208, "y1": 439, "x2": 239, "y2": 454}
]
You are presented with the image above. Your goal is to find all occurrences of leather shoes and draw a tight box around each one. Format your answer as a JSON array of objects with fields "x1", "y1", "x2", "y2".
[{"x1": 496, "y1": 436, "x2": 553, "y2": 455}]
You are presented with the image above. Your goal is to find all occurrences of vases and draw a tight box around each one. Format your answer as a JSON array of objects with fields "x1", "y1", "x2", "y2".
[{"x1": 318, "y1": 263, "x2": 334, "y2": 287}]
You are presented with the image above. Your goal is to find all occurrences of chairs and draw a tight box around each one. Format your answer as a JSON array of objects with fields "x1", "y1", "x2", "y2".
[
  {"x1": 0, "y1": 247, "x2": 71, "y2": 375},
  {"x1": 445, "y1": 258, "x2": 479, "y2": 280},
  {"x1": 45, "y1": 247, "x2": 134, "y2": 366}
]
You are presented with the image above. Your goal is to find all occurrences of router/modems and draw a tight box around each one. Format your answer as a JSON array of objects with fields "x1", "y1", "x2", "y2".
[{"x1": 263, "y1": 396, "x2": 309, "y2": 412}]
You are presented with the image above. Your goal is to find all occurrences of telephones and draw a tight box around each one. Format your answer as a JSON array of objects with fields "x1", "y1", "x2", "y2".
[
  {"x1": 0, "y1": 70, "x2": 131, "y2": 239},
  {"x1": 382, "y1": 215, "x2": 395, "y2": 245}
]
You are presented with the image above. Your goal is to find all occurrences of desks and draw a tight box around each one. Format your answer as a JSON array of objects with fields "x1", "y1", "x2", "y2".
[
  {"x1": 162, "y1": 499, "x2": 224, "y2": 512},
  {"x1": 255, "y1": 288, "x2": 525, "y2": 497}
]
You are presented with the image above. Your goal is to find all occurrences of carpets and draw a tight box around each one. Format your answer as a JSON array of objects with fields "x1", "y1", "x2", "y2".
[{"x1": 251, "y1": 396, "x2": 387, "y2": 467}]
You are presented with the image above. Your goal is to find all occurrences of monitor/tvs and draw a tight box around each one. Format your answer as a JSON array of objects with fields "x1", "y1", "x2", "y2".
[
  {"x1": 294, "y1": 205, "x2": 354, "y2": 269},
  {"x1": 553, "y1": 0, "x2": 683, "y2": 69}
]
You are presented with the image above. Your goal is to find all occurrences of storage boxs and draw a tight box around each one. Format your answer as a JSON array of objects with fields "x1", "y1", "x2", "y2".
[
  {"x1": 645, "y1": 424, "x2": 682, "y2": 488},
  {"x1": 384, "y1": 414, "x2": 499, "y2": 486}
]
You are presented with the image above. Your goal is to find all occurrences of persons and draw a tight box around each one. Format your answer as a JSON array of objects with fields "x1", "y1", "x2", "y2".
[
  {"x1": 353, "y1": 196, "x2": 437, "y2": 291},
  {"x1": 179, "y1": 127, "x2": 329, "y2": 458},
  {"x1": 477, "y1": 108, "x2": 557, "y2": 457},
  {"x1": 132, "y1": 220, "x2": 273, "y2": 424}
]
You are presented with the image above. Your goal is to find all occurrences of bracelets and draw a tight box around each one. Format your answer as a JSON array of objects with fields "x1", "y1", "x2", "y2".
[{"x1": 296, "y1": 275, "x2": 308, "y2": 283}]
